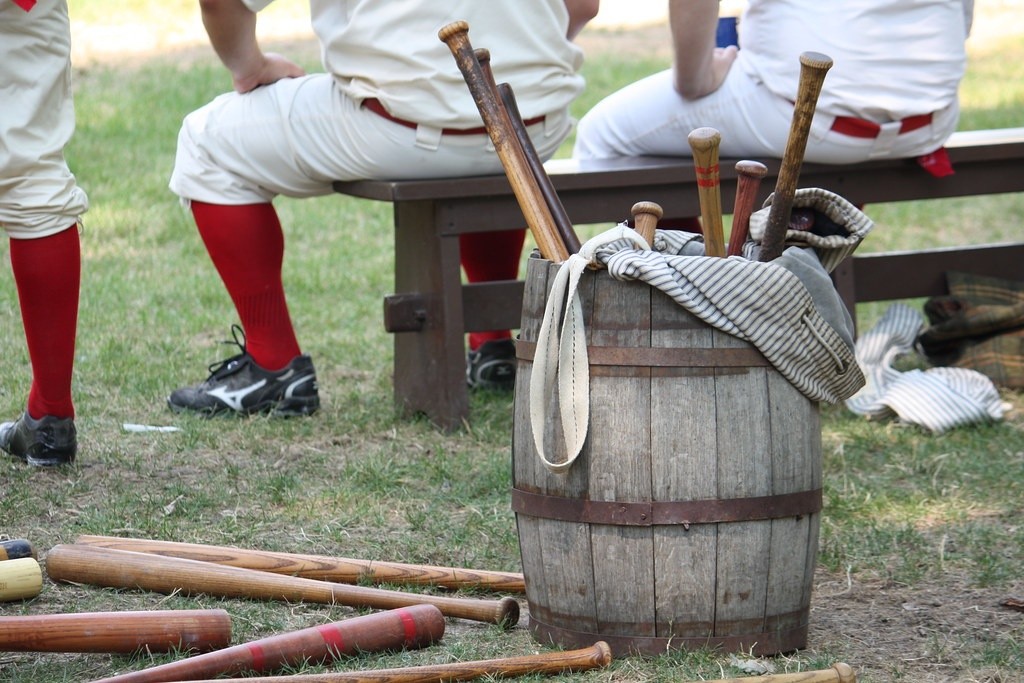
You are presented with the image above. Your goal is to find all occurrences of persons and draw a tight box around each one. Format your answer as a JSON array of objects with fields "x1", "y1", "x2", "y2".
[
  {"x1": 168, "y1": 0, "x2": 600, "y2": 419},
  {"x1": 0, "y1": 0, "x2": 90, "y2": 464},
  {"x1": 576, "y1": 1, "x2": 973, "y2": 233}
]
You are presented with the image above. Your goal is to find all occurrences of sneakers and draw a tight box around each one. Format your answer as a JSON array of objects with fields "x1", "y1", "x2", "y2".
[
  {"x1": 465, "y1": 339, "x2": 517, "y2": 391},
  {"x1": 0, "y1": 410, "x2": 78, "y2": 466},
  {"x1": 167, "y1": 324, "x2": 320, "y2": 418}
]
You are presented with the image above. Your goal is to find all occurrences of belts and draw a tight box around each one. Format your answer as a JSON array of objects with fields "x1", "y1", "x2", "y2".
[
  {"x1": 788, "y1": 100, "x2": 934, "y2": 139},
  {"x1": 360, "y1": 98, "x2": 547, "y2": 135}
]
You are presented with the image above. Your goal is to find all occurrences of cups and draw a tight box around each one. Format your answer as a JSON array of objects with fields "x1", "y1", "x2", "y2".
[{"x1": 717, "y1": 17, "x2": 739, "y2": 50}]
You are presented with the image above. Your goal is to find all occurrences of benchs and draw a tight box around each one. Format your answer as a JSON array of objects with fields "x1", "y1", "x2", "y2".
[{"x1": 333, "y1": 125, "x2": 1024, "y2": 432}]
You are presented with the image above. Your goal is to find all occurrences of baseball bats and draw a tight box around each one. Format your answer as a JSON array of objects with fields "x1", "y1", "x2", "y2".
[
  {"x1": 0, "y1": 610, "x2": 233, "y2": 648},
  {"x1": 0, "y1": 533, "x2": 43, "y2": 599},
  {"x1": 75, "y1": 532, "x2": 526, "y2": 592},
  {"x1": 437, "y1": 15, "x2": 836, "y2": 264},
  {"x1": 83, "y1": 605, "x2": 445, "y2": 682},
  {"x1": 206, "y1": 642, "x2": 612, "y2": 683},
  {"x1": 703, "y1": 663, "x2": 856, "y2": 681},
  {"x1": 42, "y1": 546, "x2": 520, "y2": 627}
]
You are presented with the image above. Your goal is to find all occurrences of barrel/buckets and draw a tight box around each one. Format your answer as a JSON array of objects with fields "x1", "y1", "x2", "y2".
[{"x1": 511, "y1": 247, "x2": 824, "y2": 656}]
